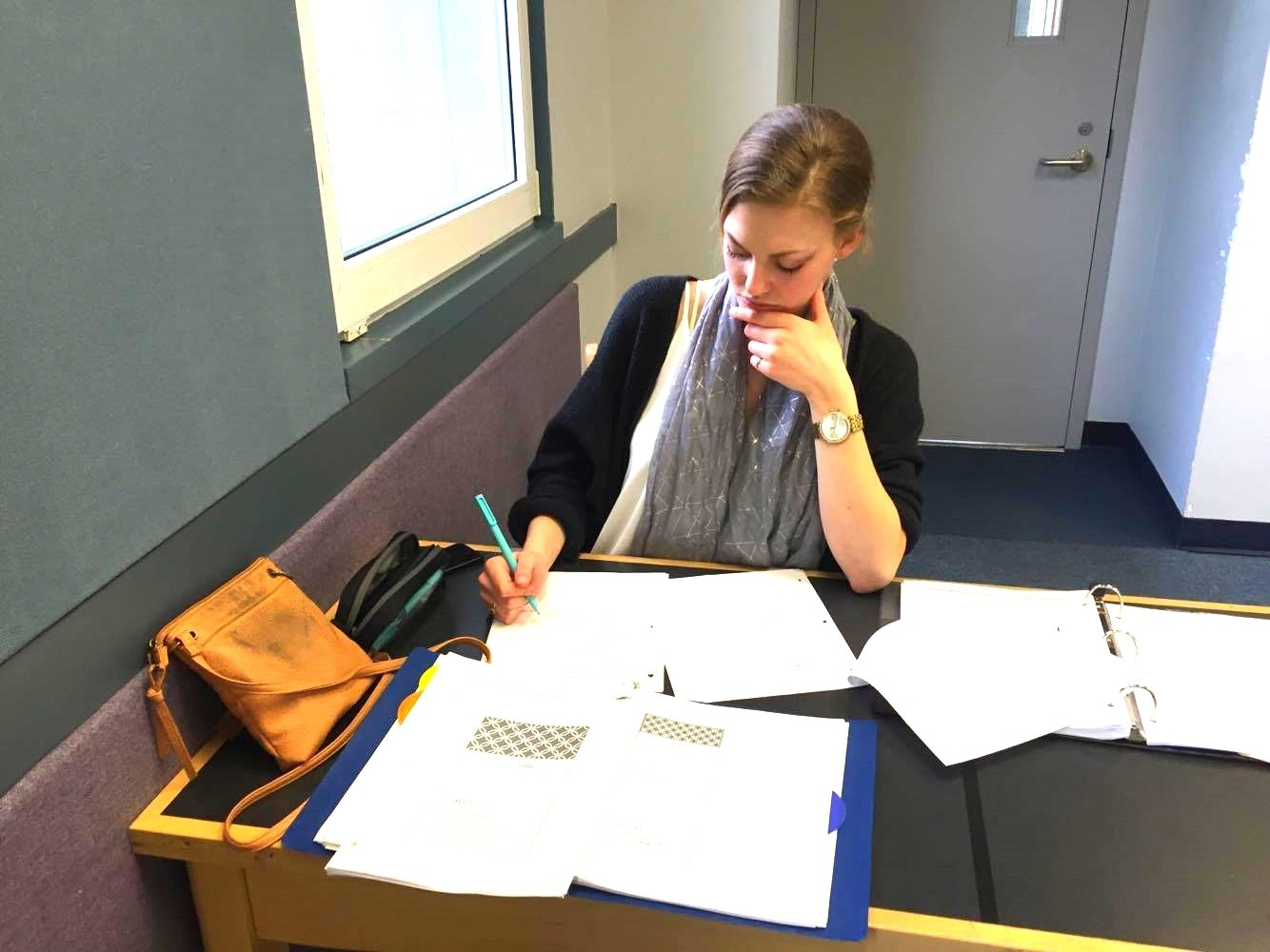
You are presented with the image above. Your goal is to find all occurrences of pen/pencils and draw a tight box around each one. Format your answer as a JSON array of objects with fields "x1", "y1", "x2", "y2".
[
  {"x1": 366, "y1": 567, "x2": 444, "y2": 658},
  {"x1": 474, "y1": 493, "x2": 542, "y2": 616}
]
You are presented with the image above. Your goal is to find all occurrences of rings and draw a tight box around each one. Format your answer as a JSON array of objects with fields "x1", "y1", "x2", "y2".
[
  {"x1": 756, "y1": 358, "x2": 763, "y2": 372},
  {"x1": 489, "y1": 601, "x2": 496, "y2": 616}
]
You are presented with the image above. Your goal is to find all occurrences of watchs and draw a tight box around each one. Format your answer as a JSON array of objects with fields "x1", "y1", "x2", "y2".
[{"x1": 810, "y1": 408, "x2": 865, "y2": 443}]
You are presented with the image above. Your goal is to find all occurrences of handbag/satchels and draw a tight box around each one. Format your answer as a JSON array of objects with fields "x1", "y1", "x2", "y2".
[
  {"x1": 144, "y1": 554, "x2": 380, "y2": 775},
  {"x1": 330, "y1": 531, "x2": 447, "y2": 656}
]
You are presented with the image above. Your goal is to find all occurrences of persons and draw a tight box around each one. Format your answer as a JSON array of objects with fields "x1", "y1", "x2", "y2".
[{"x1": 477, "y1": 102, "x2": 930, "y2": 626}]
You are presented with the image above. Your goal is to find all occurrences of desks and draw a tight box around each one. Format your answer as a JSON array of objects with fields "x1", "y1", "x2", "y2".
[{"x1": 128, "y1": 541, "x2": 1270, "y2": 951}]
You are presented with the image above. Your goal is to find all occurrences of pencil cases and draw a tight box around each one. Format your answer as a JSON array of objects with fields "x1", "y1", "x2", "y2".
[{"x1": 330, "y1": 528, "x2": 498, "y2": 666}]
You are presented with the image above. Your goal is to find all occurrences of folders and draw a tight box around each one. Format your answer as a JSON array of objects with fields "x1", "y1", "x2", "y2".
[
  {"x1": 871, "y1": 576, "x2": 1270, "y2": 767},
  {"x1": 277, "y1": 646, "x2": 876, "y2": 943}
]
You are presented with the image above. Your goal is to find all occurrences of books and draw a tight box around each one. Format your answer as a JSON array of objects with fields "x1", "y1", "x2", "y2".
[
  {"x1": 844, "y1": 573, "x2": 1269, "y2": 769},
  {"x1": 289, "y1": 649, "x2": 884, "y2": 941}
]
